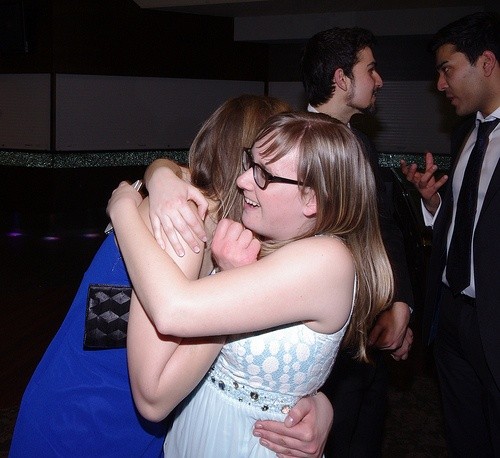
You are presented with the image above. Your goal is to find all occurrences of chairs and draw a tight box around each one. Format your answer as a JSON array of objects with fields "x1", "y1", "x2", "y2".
[{"x1": 389, "y1": 166, "x2": 433, "y2": 349}]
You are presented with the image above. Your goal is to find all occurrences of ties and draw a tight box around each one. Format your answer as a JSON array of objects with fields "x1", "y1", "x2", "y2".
[{"x1": 445, "y1": 118, "x2": 499, "y2": 296}]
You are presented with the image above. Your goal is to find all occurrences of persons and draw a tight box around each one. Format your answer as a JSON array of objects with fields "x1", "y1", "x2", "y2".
[
  {"x1": 302, "y1": 28, "x2": 417, "y2": 458},
  {"x1": 102, "y1": 110, "x2": 395, "y2": 458},
  {"x1": 397, "y1": 16, "x2": 499, "y2": 458},
  {"x1": 4, "y1": 93, "x2": 343, "y2": 458}
]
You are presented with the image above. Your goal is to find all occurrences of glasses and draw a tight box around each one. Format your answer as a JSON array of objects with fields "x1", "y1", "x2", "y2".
[{"x1": 241, "y1": 146, "x2": 309, "y2": 191}]
